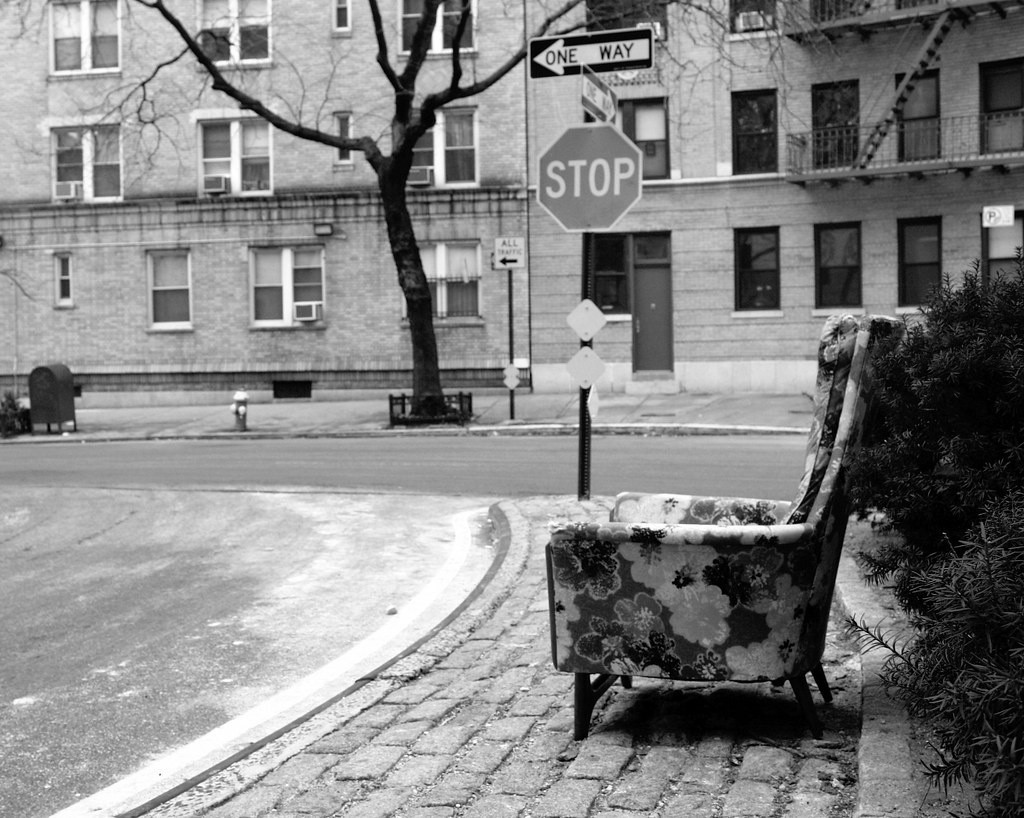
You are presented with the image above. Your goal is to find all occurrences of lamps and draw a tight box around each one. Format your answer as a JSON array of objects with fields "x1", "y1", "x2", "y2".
[{"x1": 312, "y1": 219, "x2": 335, "y2": 237}]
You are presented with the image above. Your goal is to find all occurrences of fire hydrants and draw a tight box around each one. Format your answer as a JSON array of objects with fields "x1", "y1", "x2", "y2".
[{"x1": 229, "y1": 389, "x2": 249, "y2": 431}]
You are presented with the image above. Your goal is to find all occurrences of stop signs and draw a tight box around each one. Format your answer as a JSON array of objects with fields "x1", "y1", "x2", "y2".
[{"x1": 536, "y1": 124, "x2": 644, "y2": 232}]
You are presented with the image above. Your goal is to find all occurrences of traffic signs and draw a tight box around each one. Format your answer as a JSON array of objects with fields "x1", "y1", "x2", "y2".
[
  {"x1": 580, "y1": 61, "x2": 617, "y2": 125},
  {"x1": 528, "y1": 26, "x2": 654, "y2": 79}
]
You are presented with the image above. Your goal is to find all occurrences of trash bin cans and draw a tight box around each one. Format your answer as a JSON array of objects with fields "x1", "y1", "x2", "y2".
[{"x1": 29, "y1": 365, "x2": 77, "y2": 434}]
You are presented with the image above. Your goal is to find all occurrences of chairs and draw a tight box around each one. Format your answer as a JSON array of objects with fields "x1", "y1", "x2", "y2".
[{"x1": 544, "y1": 312, "x2": 907, "y2": 740}]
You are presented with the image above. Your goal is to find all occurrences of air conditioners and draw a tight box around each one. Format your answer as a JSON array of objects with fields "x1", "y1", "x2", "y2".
[
  {"x1": 295, "y1": 300, "x2": 322, "y2": 321},
  {"x1": 404, "y1": 165, "x2": 436, "y2": 187},
  {"x1": 740, "y1": 11, "x2": 766, "y2": 33},
  {"x1": 54, "y1": 180, "x2": 82, "y2": 200},
  {"x1": 635, "y1": 21, "x2": 666, "y2": 41},
  {"x1": 203, "y1": 173, "x2": 229, "y2": 193}
]
